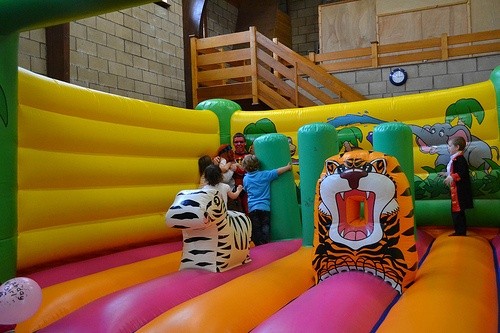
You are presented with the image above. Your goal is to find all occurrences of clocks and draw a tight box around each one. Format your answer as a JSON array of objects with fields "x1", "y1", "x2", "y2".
[{"x1": 389, "y1": 67, "x2": 408, "y2": 86}]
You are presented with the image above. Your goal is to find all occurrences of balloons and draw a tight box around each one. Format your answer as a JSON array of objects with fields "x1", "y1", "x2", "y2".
[{"x1": 0, "y1": 277, "x2": 42, "y2": 325}]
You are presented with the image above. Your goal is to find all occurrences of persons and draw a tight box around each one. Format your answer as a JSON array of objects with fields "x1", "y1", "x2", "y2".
[
  {"x1": 440, "y1": 136, "x2": 473, "y2": 236},
  {"x1": 199, "y1": 133, "x2": 292, "y2": 245}
]
